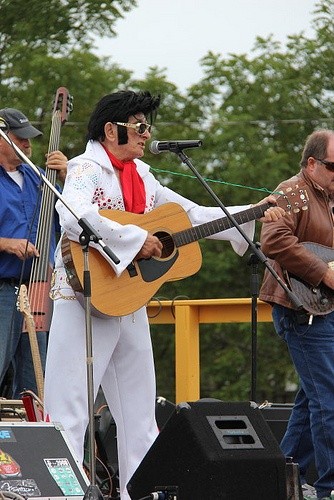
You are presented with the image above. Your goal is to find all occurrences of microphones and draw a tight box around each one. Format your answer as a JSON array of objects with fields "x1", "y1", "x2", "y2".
[{"x1": 148, "y1": 140, "x2": 203, "y2": 155}]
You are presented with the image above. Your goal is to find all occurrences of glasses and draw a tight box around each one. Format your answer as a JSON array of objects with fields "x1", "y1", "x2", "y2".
[
  {"x1": 112, "y1": 122, "x2": 151, "y2": 135},
  {"x1": 315, "y1": 158, "x2": 334, "y2": 171}
]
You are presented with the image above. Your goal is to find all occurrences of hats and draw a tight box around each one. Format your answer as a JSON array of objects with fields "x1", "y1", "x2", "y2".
[{"x1": 0, "y1": 107, "x2": 43, "y2": 139}]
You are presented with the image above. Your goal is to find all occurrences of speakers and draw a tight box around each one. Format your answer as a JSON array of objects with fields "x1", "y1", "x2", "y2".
[{"x1": 126, "y1": 401, "x2": 287, "y2": 500}]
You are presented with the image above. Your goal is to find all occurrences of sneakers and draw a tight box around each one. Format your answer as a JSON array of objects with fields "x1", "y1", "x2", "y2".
[{"x1": 302, "y1": 483, "x2": 334, "y2": 500}]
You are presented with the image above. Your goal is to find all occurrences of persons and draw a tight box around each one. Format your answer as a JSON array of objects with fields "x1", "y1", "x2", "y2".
[
  {"x1": 44, "y1": 90, "x2": 286, "y2": 500},
  {"x1": 259, "y1": 129, "x2": 334, "y2": 500},
  {"x1": 0, "y1": 107, "x2": 68, "y2": 400}
]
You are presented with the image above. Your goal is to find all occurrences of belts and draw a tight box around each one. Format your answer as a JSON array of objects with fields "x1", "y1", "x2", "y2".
[{"x1": 49, "y1": 268, "x2": 77, "y2": 301}]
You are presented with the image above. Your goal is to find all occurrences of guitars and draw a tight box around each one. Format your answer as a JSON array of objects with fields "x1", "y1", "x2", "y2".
[
  {"x1": 61, "y1": 184, "x2": 309, "y2": 320},
  {"x1": 283, "y1": 242, "x2": 334, "y2": 316},
  {"x1": 16, "y1": 285, "x2": 49, "y2": 422}
]
